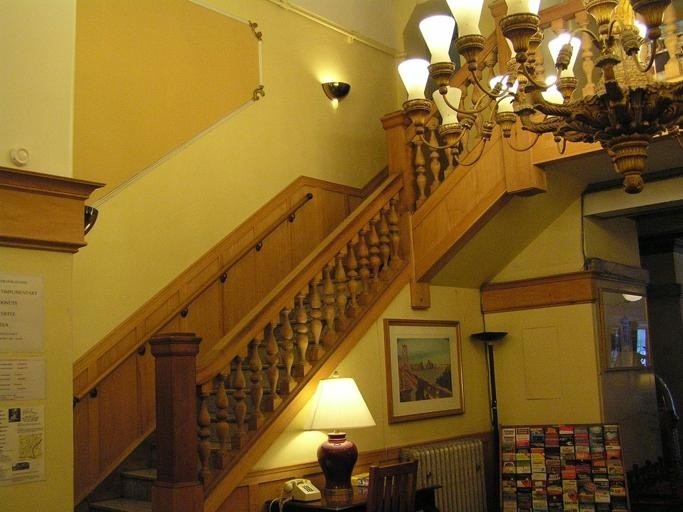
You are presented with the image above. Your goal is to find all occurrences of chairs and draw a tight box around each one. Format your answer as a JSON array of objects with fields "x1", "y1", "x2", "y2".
[{"x1": 366, "y1": 459, "x2": 418, "y2": 512}]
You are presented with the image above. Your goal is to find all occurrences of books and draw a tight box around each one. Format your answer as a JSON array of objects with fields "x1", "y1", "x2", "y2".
[{"x1": 500, "y1": 425, "x2": 628, "y2": 512}]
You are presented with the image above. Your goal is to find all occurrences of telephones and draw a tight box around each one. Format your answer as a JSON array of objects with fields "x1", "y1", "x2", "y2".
[{"x1": 278, "y1": 478, "x2": 322, "y2": 503}]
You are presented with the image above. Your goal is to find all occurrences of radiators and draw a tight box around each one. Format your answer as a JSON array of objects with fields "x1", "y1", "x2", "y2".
[{"x1": 399, "y1": 437, "x2": 488, "y2": 512}]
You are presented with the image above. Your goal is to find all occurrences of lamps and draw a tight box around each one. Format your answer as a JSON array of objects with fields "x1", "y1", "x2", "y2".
[
  {"x1": 302, "y1": 378, "x2": 376, "y2": 489},
  {"x1": 469, "y1": 332, "x2": 509, "y2": 512},
  {"x1": 321, "y1": 82, "x2": 351, "y2": 101},
  {"x1": 397, "y1": 0, "x2": 683, "y2": 195}
]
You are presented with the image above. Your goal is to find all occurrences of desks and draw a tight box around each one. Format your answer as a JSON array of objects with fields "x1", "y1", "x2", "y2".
[{"x1": 264, "y1": 478, "x2": 442, "y2": 512}]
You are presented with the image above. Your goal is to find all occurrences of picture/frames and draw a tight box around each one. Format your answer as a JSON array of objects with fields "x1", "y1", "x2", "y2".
[
  {"x1": 383, "y1": 317, "x2": 464, "y2": 425},
  {"x1": 598, "y1": 287, "x2": 654, "y2": 372}
]
